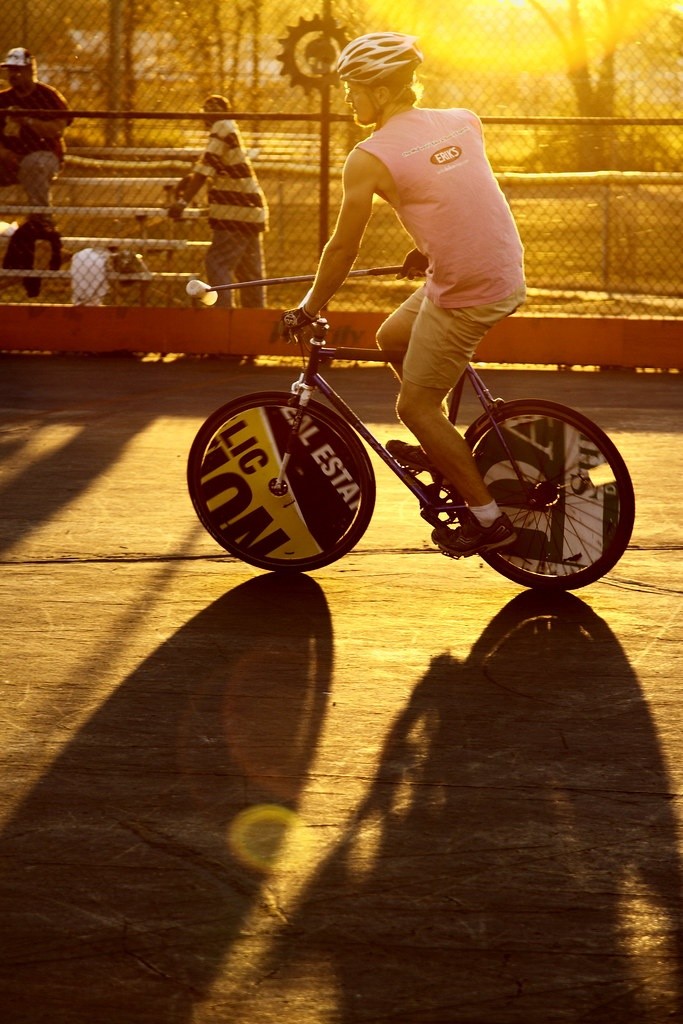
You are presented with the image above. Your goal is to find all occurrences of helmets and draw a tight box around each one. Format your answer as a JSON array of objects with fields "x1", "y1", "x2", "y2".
[{"x1": 337, "y1": 31, "x2": 423, "y2": 86}]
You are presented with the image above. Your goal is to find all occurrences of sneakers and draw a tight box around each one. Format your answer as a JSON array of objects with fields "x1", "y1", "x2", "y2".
[
  {"x1": 432, "y1": 510, "x2": 516, "y2": 555},
  {"x1": 386, "y1": 439, "x2": 441, "y2": 478}
]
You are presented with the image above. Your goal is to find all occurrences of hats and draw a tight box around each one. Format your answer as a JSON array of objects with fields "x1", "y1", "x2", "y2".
[{"x1": 0, "y1": 46, "x2": 33, "y2": 67}]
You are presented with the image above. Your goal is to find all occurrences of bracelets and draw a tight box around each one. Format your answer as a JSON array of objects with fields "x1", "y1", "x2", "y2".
[
  {"x1": 25, "y1": 118, "x2": 32, "y2": 126},
  {"x1": 177, "y1": 198, "x2": 188, "y2": 207}
]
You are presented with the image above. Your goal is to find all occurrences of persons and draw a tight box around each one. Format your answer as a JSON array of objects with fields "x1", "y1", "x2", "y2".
[
  {"x1": 0, "y1": 47, "x2": 76, "y2": 240},
  {"x1": 263, "y1": 32, "x2": 526, "y2": 557},
  {"x1": 167, "y1": 96, "x2": 270, "y2": 309}
]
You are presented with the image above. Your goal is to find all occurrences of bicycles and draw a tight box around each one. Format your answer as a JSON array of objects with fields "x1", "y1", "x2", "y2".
[{"x1": 186, "y1": 313, "x2": 633, "y2": 591}]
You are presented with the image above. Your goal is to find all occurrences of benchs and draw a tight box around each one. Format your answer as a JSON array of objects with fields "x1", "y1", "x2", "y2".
[{"x1": 0, "y1": 177, "x2": 212, "y2": 307}]
